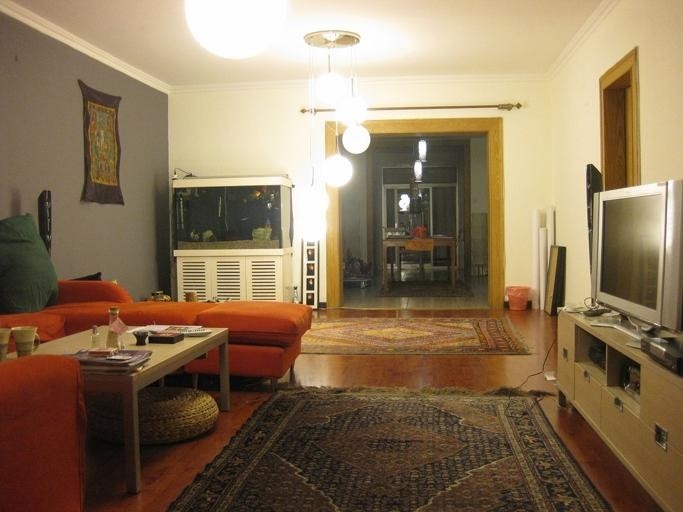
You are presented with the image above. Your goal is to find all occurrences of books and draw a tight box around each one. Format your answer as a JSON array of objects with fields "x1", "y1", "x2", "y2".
[{"x1": 71, "y1": 347, "x2": 155, "y2": 377}]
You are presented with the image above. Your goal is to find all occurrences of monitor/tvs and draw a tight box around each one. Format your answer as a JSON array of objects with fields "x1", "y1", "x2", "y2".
[{"x1": 590, "y1": 178, "x2": 682, "y2": 332}]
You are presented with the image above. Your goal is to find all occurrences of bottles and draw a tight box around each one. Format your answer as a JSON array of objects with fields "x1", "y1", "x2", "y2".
[
  {"x1": 89, "y1": 325, "x2": 99, "y2": 346},
  {"x1": 105, "y1": 303, "x2": 125, "y2": 350},
  {"x1": 290, "y1": 285, "x2": 299, "y2": 303}
]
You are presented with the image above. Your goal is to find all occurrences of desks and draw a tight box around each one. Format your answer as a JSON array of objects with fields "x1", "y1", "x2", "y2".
[{"x1": 382, "y1": 233, "x2": 457, "y2": 292}]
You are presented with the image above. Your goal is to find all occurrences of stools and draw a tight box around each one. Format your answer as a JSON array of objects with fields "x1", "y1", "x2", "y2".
[{"x1": 182, "y1": 300, "x2": 313, "y2": 396}]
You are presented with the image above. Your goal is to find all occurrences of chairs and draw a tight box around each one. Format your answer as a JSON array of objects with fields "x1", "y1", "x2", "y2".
[{"x1": 406, "y1": 237, "x2": 440, "y2": 285}]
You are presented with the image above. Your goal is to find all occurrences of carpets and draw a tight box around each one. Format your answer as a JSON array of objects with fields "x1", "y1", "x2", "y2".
[
  {"x1": 167, "y1": 383, "x2": 613, "y2": 511},
  {"x1": 375, "y1": 280, "x2": 474, "y2": 298},
  {"x1": 294, "y1": 316, "x2": 530, "y2": 355}
]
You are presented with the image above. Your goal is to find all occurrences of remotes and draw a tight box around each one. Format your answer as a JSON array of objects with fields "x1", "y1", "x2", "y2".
[
  {"x1": 584, "y1": 309, "x2": 612, "y2": 316},
  {"x1": 157, "y1": 329, "x2": 212, "y2": 337}
]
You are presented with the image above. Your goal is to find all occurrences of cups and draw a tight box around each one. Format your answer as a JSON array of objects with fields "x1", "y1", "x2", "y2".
[
  {"x1": 12, "y1": 325, "x2": 41, "y2": 357},
  {"x1": 183, "y1": 290, "x2": 197, "y2": 302},
  {"x1": 0, "y1": 328, "x2": 9, "y2": 362}
]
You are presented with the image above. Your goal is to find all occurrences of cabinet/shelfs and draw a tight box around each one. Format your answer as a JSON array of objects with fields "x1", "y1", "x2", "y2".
[{"x1": 554, "y1": 303, "x2": 683, "y2": 511}]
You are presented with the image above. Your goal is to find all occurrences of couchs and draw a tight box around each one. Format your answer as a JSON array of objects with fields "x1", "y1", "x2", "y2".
[{"x1": 0, "y1": 280, "x2": 218, "y2": 510}]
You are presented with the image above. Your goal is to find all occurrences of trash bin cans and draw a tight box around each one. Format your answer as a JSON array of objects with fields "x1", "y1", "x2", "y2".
[{"x1": 507, "y1": 286, "x2": 530, "y2": 311}]
